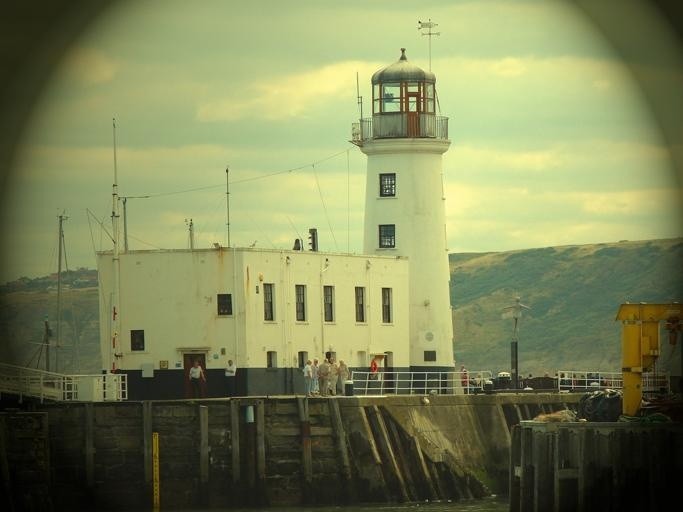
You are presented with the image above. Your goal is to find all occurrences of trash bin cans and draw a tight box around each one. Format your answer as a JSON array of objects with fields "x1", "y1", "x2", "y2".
[
  {"x1": 485, "y1": 380, "x2": 493, "y2": 394},
  {"x1": 497, "y1": 371, "x2": 510, "y2": 389},
  {"x1": 344, "y1": 380, "x2": 353, "y2": 396}
]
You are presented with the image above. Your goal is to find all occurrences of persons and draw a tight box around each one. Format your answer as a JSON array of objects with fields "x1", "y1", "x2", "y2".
[
  {"x1": 521, "y1": 370, "x2": 608, "y2": 388},
  {"x1": 326, "y1": 357, "x2": 338, "y2": 395},
  {"x1": 316, "y1": 358, "x2": 329, "y2": 396},
  {"x1": 300, "y1": 359, "x2": 312, "y2": 397},
  {"x1": 310, "y1": 357, "x2": 318, "y2": 395},
  {"x1": 222, "y1": 359, "x2": 236, "y2": 395},
  {"x1": 458, "y1": 364, "x2": 468, "y2": 394},
  {"x1": 333, "y1": 359, "x2": 349, "y2": 395},
  {"x1": 188, "y1": 360, "x2": 206, "y2": 397}
]
